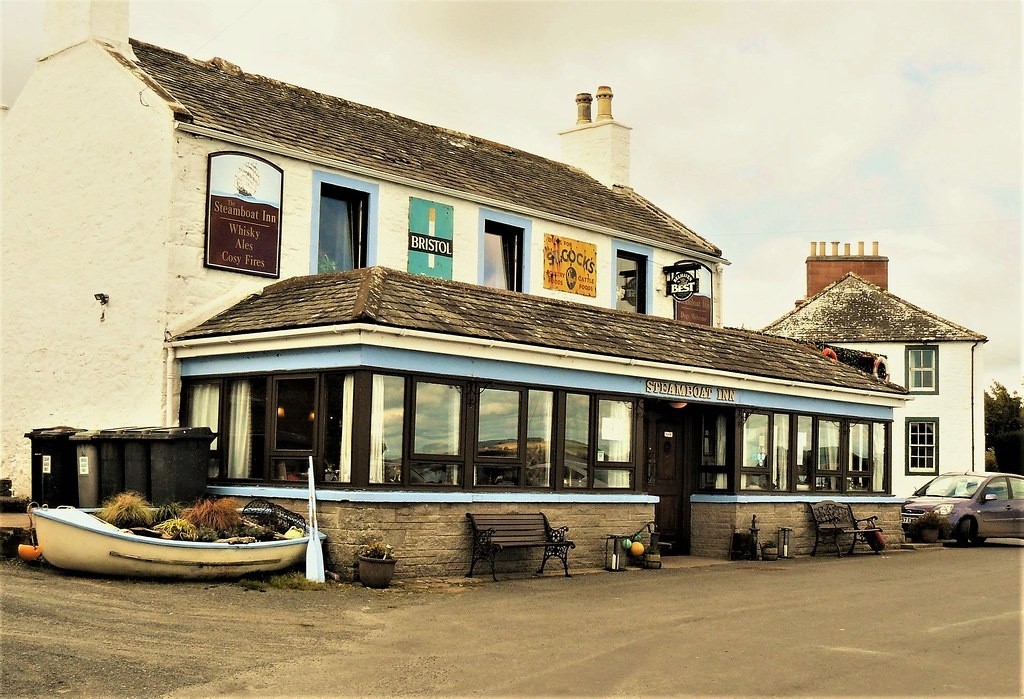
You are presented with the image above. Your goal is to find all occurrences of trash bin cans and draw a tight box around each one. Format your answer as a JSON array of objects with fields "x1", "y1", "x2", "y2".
[
  {"x1": 35, "y1": 429, "x2": 89, "y2": 508},
  {"x1": 118, "y1": 427, "x2": 179, "y2": 505},
  {"x1": 69, "y1": 429, "x2": 103, "y2": 508},
  {"x1": 24, "y1": 426, "x2": 74, "y2": 508},
  {"x1": 92, "y1": 426, "x2": 138, "y2": 508},
  {"x1": 142, "y1": 427, "x2": 221, "y2": 508}
]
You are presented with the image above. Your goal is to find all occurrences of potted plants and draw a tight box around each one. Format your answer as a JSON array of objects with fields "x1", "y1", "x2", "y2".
[
  {"x1": 357, "y1": 538, "x2": 397, "y2": 590},
  {"x1": 761, "y1": 540, "x2": 778, "y2": 561},
  {"x1": 642, "y1": 545, "x2": 661, "y2": 569}
]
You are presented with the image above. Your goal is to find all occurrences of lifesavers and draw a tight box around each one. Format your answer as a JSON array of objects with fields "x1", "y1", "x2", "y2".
[
  {"x1": 873, "y1": 356, "x2": 890, "y2": 383},
  {"x1": 821, "y1": 348, "x2": 838, "y2": 361}
]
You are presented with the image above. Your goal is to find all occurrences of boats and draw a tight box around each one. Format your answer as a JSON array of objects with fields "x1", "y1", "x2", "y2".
[{"x1": 18, "y1": 500, "x2": 324, "y2": 581}]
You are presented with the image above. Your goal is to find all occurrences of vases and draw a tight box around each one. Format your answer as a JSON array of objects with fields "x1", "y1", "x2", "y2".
[{"x1": 920, "y1": 528, "x2": 940, "y2": 542}]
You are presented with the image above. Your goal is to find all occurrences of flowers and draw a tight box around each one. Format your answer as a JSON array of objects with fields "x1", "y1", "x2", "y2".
[{"x1": 906, "y1": 508, "x2": 952, "y2": 540}]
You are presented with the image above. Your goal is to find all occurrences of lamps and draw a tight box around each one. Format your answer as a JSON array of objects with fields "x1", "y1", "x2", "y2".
[
  {"x1": 669, "y1": 402, "x2": 688, "y2": 408},
  {"x1": 94, "y1": 293, "x2": 108, "y2": 304}
]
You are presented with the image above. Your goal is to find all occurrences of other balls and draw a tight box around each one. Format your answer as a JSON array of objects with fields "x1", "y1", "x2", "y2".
[{"x1": 622, "y1": 534, "x2": 644, "y2": 555}]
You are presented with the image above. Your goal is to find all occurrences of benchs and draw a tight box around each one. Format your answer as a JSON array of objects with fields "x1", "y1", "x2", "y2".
[
  {"x1": 465, "y1": 512, "x2": 575, "y2": 581},
  {"x1": 807, "y1": 500, "x2": 883, "y2": 559}
]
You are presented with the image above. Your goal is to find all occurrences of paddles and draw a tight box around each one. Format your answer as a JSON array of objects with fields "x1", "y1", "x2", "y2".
[
  {"x1": 308, "y1": 456, "x2": 325, "y2": 584},
  {"x1": 306, "y1": 467, "x2": 319, "y2": 581}
]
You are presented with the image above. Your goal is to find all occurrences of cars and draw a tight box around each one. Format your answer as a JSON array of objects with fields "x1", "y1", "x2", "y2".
[{"x1": 901, "y1": 472, "x2": 1024, "y2": 548}]
